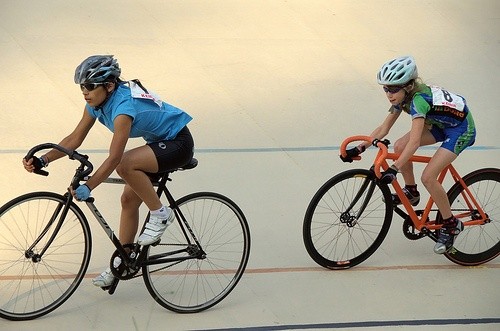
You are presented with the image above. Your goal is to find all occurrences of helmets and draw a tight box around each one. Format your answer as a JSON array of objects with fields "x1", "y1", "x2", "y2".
[
  {"x1": 74, "y1": 54, "x2": 122, "y2": 84},
  {"x1": 376, "y1": 55, "x2": 419, "y2": 87}
]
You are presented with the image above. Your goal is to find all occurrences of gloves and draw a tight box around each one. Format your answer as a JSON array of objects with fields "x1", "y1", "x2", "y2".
[
  {"x1": 378, "y1": 165, "x2": 398, "y2": 186},
  {"x1": 339, "y1": 147, "x2": 360, "y2": 164}
]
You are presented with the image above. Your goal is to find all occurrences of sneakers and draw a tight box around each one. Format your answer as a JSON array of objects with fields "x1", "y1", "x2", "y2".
[
  {"x1": 433, "y1": 219, "x2": 464, "y2": 254},
  {"x1": 137, "y1": 207, "x2": 175, "y2": 245},
  {"x1": 92, "y1": 264, "x2": 142, "y2": 286},
  {"x1": 381, "y1": 188, "x2": 421, "y2": 206}
]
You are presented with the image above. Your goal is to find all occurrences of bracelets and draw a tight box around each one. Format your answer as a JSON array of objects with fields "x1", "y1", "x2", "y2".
[{"x1": 42, "y1": 155, "x2": 49, "y2": 165}]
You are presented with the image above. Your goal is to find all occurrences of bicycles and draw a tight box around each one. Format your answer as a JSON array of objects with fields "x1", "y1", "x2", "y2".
[
  {"x1": 0, "y1": 143, "x2": 251, "y2": 322},
  {"x1": 302, "y1": 135, "x2": 500, "y2": 271}
]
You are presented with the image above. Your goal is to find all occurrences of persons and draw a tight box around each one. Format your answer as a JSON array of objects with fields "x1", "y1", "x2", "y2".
[
  {"x1": 339, "y1": 57, "x2": 476, "y2": 254},
  {"x1": 21, "y1": 55, "x2": 194, "y2": 286}
]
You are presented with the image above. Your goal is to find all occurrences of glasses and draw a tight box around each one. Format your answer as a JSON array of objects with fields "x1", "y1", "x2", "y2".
[
  {"x1": 79, "y1": 82, "x2": 109, "y2": 90},
  {"x1": 382, "y1": 84, "x2": 411, "y2": 93}
]
